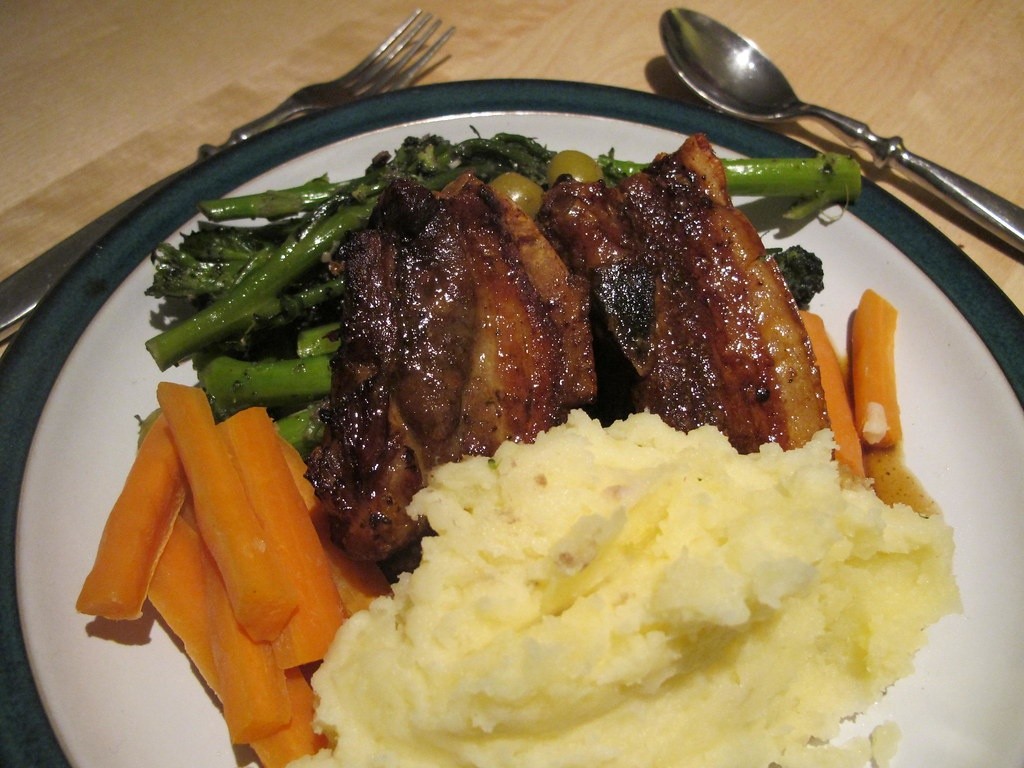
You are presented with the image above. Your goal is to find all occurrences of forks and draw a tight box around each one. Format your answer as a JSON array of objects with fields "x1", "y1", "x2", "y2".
[{"x1": 1, "y1": 8, "x2": 456, "y2": 331}]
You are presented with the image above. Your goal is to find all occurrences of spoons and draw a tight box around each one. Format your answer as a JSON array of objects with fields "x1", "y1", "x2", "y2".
[{"x1": 659, "y1": 7, "x2": 1024, "y2": 254}]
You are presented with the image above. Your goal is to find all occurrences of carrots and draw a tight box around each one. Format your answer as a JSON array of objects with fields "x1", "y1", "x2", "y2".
[
  {"x1": 74, "y1": 381, "x2": 396, "y2": 768},
  {"x1": 795, "y1": 288, "x2": 902, "y2": 480}
]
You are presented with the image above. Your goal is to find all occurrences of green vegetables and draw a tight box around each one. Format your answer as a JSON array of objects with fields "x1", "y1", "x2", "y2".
[{"x1": 143, "y1": 131, "x2": 862, "y2": 461}]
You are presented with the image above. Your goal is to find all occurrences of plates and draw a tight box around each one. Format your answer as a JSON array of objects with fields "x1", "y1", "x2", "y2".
[{"x1": 0, "y1": 78, "x2": 1024, "y2": 768}]
